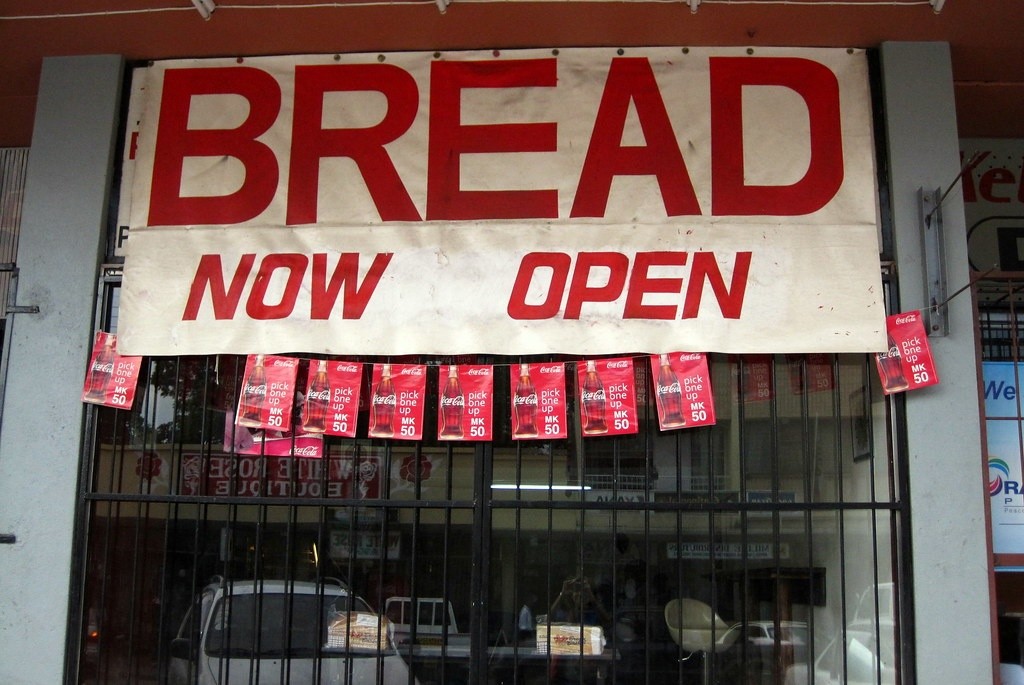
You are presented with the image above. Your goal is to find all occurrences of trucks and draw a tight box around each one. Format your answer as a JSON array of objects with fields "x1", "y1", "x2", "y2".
[{"x1": 386, "y1": 597, "x2": 472, "y2": 659}]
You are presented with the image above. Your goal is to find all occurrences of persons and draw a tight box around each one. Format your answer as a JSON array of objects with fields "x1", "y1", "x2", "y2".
[
  {"x1": 546, "y1": 577, "x2": 636, "y2": 685},
  {"x1": 599, "y1": 533, "x2": 640, "y2": 584},
  {"x1": 519, "y1": 598, "x2": 537, "y2": 642}
]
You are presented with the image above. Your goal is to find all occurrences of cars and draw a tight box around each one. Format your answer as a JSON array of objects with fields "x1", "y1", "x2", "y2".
[
  {"x1": 686, "y1": 621, "x2": 808, "y2": 685},
  {"x1": 786, "y1": 632, "x2": 896, "y2": 685},
  {"x1": 847, "y1": 583, "x2": 893, "y2": 630}
]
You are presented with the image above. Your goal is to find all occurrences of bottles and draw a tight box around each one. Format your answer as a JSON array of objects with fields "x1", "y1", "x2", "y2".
[
  {"x1": 84, "y1": 334, "x2": 116, "y2": 404},
  {"x1": 581, "y1": 361, "x2": 608, "y2": 435},
  {"x1": 239, "y1": 354, "x2": 267, "y2": 427},
  {"x1": 303, "y1": 360, "x2": 330, "y2": 433},
  {"x1": 657, "y1": 354, "x2": 686, "y2": 428},
  {"x1": 514, "y1": 364, "x2": 539, "y2": 437},
  {"x1": 370, "y1": 364, "x2": 396, "y2": 437},
  {"x1": 877, "y1": 331, "x2": 909, "y2": 393},
  {"x1": 440, "y1": 365, "x2": 465, "y2": 439}
]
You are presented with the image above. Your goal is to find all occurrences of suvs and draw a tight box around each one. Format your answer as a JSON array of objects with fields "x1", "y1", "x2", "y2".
[{"x1": 167, "y1": 575, "x2": 421, "y2": 685}]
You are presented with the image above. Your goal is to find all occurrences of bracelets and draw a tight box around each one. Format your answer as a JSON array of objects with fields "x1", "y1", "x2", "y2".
[{"x1": 560, "y1": 592, "x2": 564, "y2": 596}]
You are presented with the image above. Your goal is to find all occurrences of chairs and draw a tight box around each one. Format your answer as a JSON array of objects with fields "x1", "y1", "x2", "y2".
[{"x1": 664, "y1": 598, "x2": 742, "y2": 685}]
